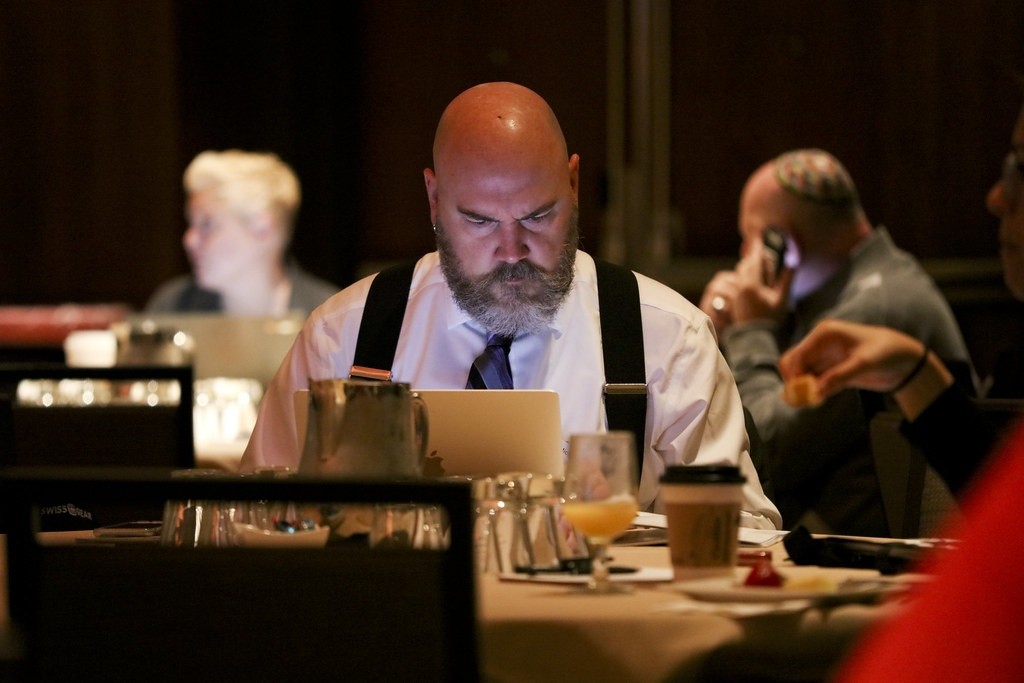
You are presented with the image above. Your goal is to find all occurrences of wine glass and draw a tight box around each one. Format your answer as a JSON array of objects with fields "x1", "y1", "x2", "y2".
[{"x1": 560, "y1": 434, "x2": 638, "y2": 592}]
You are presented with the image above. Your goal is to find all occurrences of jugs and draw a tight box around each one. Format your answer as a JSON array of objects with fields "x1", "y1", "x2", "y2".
[{"x1": 295, "y1": 379, "x2": 429, "y2": 479}]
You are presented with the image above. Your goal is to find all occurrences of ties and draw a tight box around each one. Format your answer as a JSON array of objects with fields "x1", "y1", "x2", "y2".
[{"x1": 464, "y1": 328, "x2": 515, "y2": 389}]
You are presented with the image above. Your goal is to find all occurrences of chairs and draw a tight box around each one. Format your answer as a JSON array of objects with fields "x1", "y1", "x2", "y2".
[
  {"x1": 770, "y1": 409, "x2": 925, "y2": 537},
  {"x1": 0, "y1": 468, "x2": 486, "y2": 683},
  {"x1": 0, "y1": 364, "x2": 196, "y2": 469}
]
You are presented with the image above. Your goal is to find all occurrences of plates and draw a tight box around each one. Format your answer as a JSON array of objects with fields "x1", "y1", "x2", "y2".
[{"x1": 676, "y1": 567, "x2": 881, "y2": 600}]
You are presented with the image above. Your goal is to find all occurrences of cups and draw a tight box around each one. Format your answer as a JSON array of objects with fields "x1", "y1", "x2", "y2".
[
  {"x1": 656, "y1": 464, "x2": 748, "y2": 579},
  {"x1": 388, "y1": 478, "x2": 590, "y2": 572},
  {"x1": 19, "y1": 378, "x2": 292, "y2": 548}
]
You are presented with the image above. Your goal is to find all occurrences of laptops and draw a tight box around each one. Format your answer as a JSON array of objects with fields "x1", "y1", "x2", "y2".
[
  {"x1": 122, "y1": 314, "x2": 302, "y2": 396},
  {"x1": 289, "y1": 388, "x2": 564, "y2": 483}
]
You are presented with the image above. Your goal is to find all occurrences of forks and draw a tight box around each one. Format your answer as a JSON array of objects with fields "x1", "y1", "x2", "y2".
[{"x1": 811, "y1": 534, "x2": 962, "y2": 549}]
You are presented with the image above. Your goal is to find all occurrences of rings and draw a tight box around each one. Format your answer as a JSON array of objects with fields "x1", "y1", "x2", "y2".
[{"x1": 713, "y1": 296, "x2": 725, "y2": 311}]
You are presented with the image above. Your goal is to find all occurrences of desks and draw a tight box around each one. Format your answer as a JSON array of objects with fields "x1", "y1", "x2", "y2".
[{"x1": 0, "y1": 510, "x2": 946, "y2": 683}]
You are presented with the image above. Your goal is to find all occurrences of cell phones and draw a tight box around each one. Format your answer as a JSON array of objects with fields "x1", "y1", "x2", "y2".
[{"x1": 761, "y1": 225, "x2": 787, "y2": 289}]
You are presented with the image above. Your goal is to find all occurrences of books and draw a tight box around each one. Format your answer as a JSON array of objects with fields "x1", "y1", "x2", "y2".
[{"x1": 612, "y1": 511, "x2": 782, "y2": 548}]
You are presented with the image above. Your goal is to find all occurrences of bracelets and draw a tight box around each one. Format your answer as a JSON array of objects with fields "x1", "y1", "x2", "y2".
[{"x1": 889, "y1": 343, "x2": 931, "y2": 394}]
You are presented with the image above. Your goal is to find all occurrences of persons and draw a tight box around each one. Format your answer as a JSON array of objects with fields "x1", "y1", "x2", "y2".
[
  {"x1": 233, "y1": 84, "x2": 785, "y2": 533},
  {"x1": 142, "y1": 152, "x2": 339, "y2": 320},
  {"x1": 843, "y1": 424, "x2": 1024, "y2": 683},
  {"x1": 699, "y1": 150, "x2": 985, "y2": 446},
  {"x1": 781, "y1": 106, "x2": 1024, "y2": 514}
]
